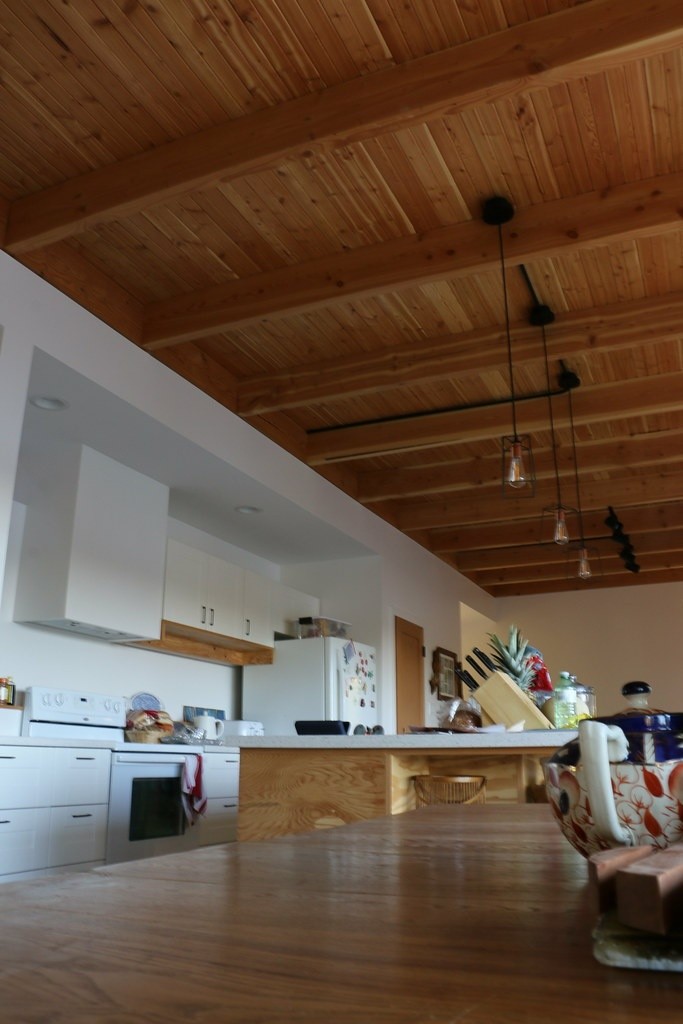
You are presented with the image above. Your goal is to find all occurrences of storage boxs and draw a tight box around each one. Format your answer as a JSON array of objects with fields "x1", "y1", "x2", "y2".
[{"x1": 292, "y1": 617, "x2": 352, "y2": 639}]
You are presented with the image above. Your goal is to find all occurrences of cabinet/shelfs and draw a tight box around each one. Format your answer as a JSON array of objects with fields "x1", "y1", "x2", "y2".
[
  {"x1": 0, "y1": 745, "x2": 112, "y2": 883},
  {"x1": 117, "y1": 538, "x2": 320, "y2": 667},
  {"x1": 198, "y1": 753, "x2": 240, "y2": 852}
]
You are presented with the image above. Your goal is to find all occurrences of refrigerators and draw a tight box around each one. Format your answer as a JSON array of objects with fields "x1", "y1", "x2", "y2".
[{"x1": 241, "y1": 637, "x2": 378, "y2": 738}]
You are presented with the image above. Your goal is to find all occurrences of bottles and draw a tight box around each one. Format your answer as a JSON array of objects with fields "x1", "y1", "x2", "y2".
[
  {"x1": 0, "y1": 678, "x2": 9, "y2": 706},
  {"x1": 6, "y1": 677, "x2": 15, "y2": 706},
  {"x1": 553, "y1": 672, "x2": 577, "y2": 728}
]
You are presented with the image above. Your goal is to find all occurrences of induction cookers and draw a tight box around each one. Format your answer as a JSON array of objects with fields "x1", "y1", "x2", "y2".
[{"x1": 20, "y1": 687, "x2": 204, "y2": 753}]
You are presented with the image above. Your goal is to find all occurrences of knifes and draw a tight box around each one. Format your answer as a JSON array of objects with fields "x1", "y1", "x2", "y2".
[{"x1": 454, "y1": 647, "x2": 497, "y2": 691}]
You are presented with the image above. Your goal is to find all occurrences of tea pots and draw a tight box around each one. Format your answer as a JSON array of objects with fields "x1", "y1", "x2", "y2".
[
  {"x1": 544, "y1": 681, "x2": 683, "y2": 860},
  {"x1": 194, "y1": 711, "x2": 223, "y2": 741}
]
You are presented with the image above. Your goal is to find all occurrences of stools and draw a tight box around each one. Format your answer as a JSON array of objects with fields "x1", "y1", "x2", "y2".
[{"x1": 414, "y1": 775, "x2": 488, "y2": 806}]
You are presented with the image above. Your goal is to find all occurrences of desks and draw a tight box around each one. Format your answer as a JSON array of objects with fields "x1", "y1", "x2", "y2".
[{"x1": 0, "y1": 804, "x2": 682, "y2": 1024}]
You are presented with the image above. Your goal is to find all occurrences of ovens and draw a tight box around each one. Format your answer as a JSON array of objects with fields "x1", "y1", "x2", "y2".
[{"x1": 105, "y1": 753, "x2": 199, "y2": 866}]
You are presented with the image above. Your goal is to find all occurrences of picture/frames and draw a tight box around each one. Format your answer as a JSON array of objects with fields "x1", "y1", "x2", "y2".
[{"x1": 436, "y1": 646, "x2": 457, "y2": 700}]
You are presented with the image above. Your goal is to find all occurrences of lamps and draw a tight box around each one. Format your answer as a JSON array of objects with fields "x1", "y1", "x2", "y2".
[
  {"x1": 606, "y1": 516, "x2": 640, "y2": 573},
  {"x1": 482, "y1": 195, "x2": 537, "y2": 500},
  {"x1": 529, "y1": 306, "x2": 583, "y2": 555},
  {"x1": 556, "y1": 372, "x2": 600, "y2": 586}
]
